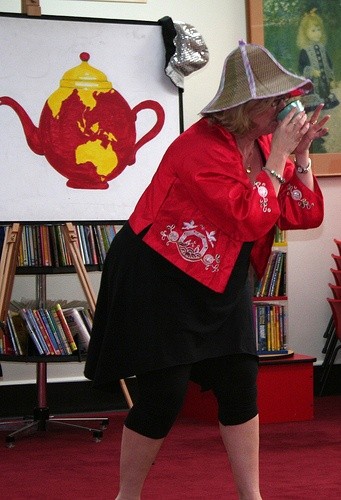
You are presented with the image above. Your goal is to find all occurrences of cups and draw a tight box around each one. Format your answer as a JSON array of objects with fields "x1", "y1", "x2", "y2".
[{"x1": 276, "y1": 99, "x2": 307, "y2": 127}]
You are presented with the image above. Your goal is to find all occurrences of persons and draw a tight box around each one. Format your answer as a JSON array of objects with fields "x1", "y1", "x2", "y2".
[{"x1": 83, "y1": 42, "x2": 330, "y2": 500}]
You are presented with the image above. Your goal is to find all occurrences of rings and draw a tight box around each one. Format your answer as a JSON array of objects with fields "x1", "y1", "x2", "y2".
[
  {"x1": 291, "y1": 121, "x2": 295, "y2": 125},
  {"x1": 309, "y1": 118, "x2": 317, "y2": 125}
]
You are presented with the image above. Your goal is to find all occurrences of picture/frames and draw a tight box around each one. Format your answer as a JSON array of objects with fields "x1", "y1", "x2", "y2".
[
  {"x1": 0, "y1": 12, "x2": 183, "y2": 224},
  {"x1": 246, "y1": 0, "x2": 341, "y2": 177}
]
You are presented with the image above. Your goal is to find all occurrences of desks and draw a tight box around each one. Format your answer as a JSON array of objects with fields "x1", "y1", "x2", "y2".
[{"x1": 182, "y1": 353, "x2": 317, "y2": 424}]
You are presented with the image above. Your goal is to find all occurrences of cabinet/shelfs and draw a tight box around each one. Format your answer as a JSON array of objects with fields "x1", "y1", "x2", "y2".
[{"x1": 0, "y1": 220, "x2": 292, "y2": 364}]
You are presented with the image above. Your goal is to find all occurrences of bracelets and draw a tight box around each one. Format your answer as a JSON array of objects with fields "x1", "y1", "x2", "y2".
[{"x1": 260, "y1": 167, "x2": 287, "y2": 185}]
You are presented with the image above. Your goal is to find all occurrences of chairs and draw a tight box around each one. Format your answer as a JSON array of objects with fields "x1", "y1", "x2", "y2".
[{"x1": 319, "y1": 239, "x2": 341, "y2": 398}]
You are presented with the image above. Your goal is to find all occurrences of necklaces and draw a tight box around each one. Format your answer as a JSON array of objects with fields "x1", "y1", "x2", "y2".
[{"x1": 237, "y1": 140, "x2": 257, "y2": 174}]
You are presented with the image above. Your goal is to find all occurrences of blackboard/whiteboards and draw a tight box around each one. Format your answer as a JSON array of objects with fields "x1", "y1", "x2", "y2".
[{"x1": 0, "y1": 12, "x2": 183, "y2": 226}]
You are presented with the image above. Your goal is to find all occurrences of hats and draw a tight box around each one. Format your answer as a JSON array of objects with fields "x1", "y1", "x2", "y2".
[
  {"x1": 159, "y1": 15, "x2": 210, "y2": 91},
  {"x1": 200, "y1": 40, "x2": 313, "y2": 115}
]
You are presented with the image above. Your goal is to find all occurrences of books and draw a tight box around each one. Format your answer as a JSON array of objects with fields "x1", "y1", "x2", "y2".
[
  {"x1": 0, "y1": 296, "x2": 95, "y2": 355},
  {"x1": 251, "y1": 302, "x2": 289, "y2": 354},
  {"x1": 252, "y1": 246, "x2": 287, "y2": 298},
  {"x1": 4, "y1": 225, "x2": 75, "y2": 266},
  {"x1": 271, "y1": 221, "x2": 288, "y2": 243},
  {"x1": 76, "y1": 225, "x2": 127, "y2": 266}
]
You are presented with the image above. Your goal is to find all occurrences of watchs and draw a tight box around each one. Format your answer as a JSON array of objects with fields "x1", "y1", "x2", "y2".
[{"x1": 294, "y1": 157, "x2": 311, "y2": 174}]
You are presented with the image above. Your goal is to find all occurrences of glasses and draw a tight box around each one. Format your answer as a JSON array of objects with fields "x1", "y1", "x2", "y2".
[{"x1": 268, "y1": 93, "x2": 291, "y2": 108}]
